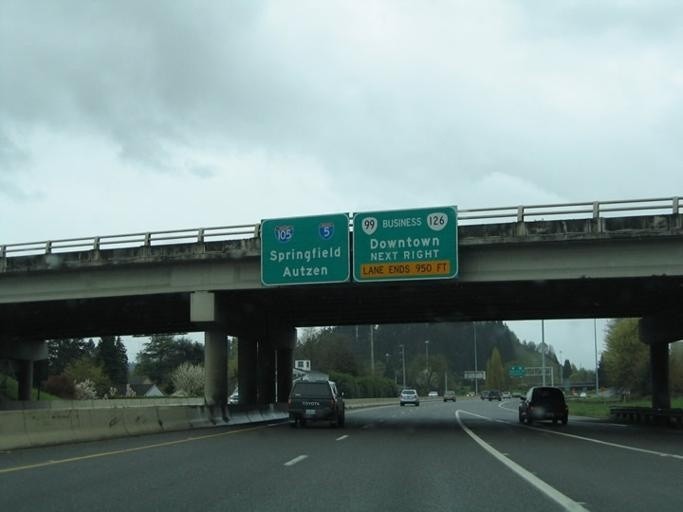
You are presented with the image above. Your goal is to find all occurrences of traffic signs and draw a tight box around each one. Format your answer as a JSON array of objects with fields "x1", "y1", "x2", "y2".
[
  {"x1": 350, "y1": 203, "x2": 459, "y2": 284},
  {"x1": 507, "y1": 365, "x2": 524, "y2": 379},
  {"x1": 258, "y1": 212, "x2": 352, "y2": 286}
]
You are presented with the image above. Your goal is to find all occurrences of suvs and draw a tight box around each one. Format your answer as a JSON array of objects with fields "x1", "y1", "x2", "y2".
[
  {"x1": 443, "y1": 390, "x2": 456, "y2": 402},
  {"x1": 287, "y1": 377, "x2": 346, "y2": 429}
]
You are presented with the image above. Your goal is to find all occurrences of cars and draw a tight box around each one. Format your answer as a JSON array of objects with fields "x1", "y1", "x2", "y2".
[
  {"x1": 573, "y1": 391, "x2": 587, "y2": 399},
  {"x1": 426, "y1": 391, "x2": 438, "y2": 397},
  {"x1": 464, "y1": 390, "x2": 520, "y2": 402},
  {"x1": 227, "y1": 385, "x2": 238, "y2": 406},
  {"x1": 399, "y1": 387, "x2": 419, "y2": 407}
]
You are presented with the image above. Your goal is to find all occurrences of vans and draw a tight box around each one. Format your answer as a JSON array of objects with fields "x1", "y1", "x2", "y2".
[{"x1": 515, "y1": 386, "x2": 568, "y2": 425}]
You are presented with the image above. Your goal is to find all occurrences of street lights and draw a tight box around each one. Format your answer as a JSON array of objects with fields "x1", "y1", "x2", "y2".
[
  {"x1": 424, "y1": 340, "x2": 429, "y2": 373},
  {"x1": 384, "y1": 353, "x2": 390, "y2": 363},
  {"x1": 558, "y1": 350, "x2": 563, "y2": 384},
  {"x1": 398, "y1": 343, "x2": 406, "y2": 388},
  {"x1": 369, "y1": 323, "x2": 379, "y2": 370}
]
